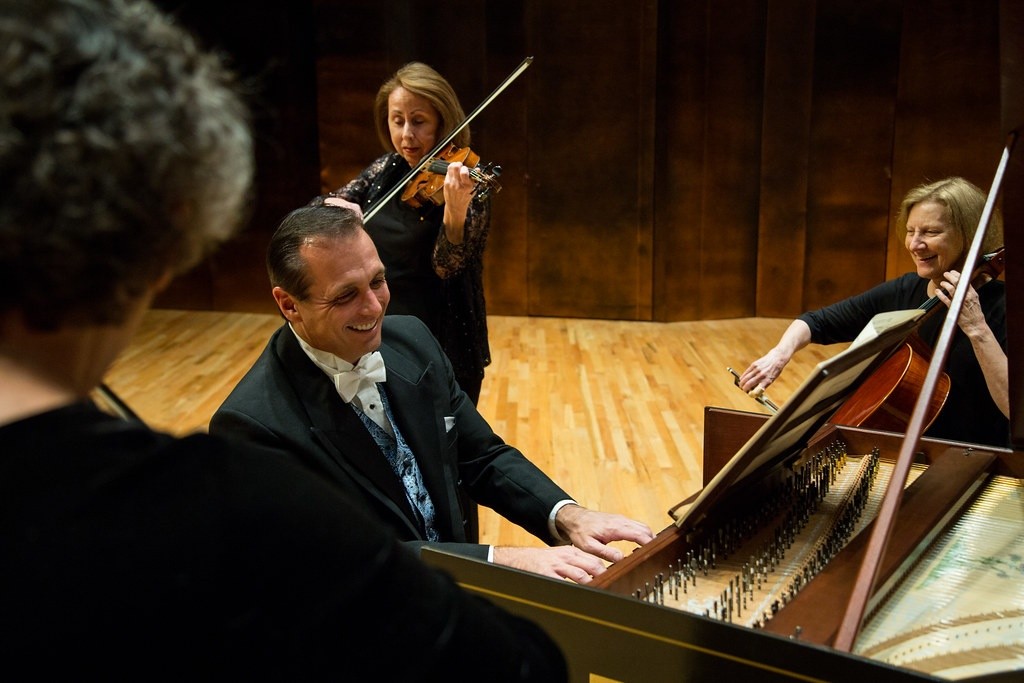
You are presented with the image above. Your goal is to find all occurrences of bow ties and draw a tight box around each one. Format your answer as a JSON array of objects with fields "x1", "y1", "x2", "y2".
[{"x1": 334, "y1": 351, "x2": 387, "y2": 402}]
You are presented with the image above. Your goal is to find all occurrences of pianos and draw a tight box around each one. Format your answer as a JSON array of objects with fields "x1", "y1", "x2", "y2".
[{"x1": 421, "y1": 408, "x2": 1023, "y2": 683}]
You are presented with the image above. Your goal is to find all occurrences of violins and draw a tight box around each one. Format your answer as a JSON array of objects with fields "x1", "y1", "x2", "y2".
[{"x1": 401, "y1": 142, "x2": 503, "y2": 212}]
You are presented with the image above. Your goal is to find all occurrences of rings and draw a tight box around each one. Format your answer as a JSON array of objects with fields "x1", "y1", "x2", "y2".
[{"x1": 758, "y1": 386, "x2": 765, "y2": 392}]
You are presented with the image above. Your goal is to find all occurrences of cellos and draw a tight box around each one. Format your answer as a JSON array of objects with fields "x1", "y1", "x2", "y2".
[{"x1": 828, "y1": 245, "x2": 1007, "y2": 438}]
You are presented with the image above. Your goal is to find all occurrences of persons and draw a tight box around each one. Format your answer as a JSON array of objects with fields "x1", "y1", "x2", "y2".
[
  {"x1": 0, "y1": 1, "x2": 571, "y2": 683},
  {"x1": 302, "y1": 62, "x2": 491, "y2": 409},
  {"x1": 209, "y1": 206, "x2": 652, "y2": 583},
  {"x1": 740, "y1": 177, "x2": 1009, "y2": 450}
]
